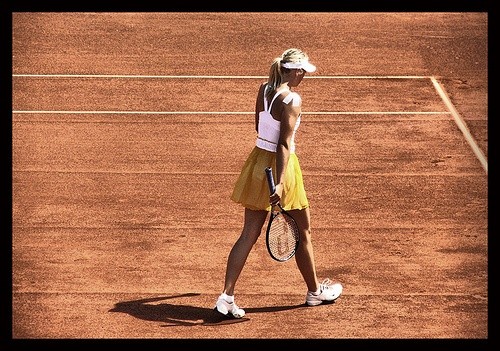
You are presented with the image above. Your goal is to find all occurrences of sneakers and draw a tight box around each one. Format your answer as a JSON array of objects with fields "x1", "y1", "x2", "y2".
[
  {"x1": 306, "y1": 278, "x2": 342, "y2": 306},
  {"x1": 215, "y1": 297, "x2": 245, "y2": 318}
]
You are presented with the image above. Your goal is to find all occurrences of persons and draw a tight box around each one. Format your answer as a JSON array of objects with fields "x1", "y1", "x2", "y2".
[{"x1": 212, "y1": 47, "x2": 343, "y2": 320}]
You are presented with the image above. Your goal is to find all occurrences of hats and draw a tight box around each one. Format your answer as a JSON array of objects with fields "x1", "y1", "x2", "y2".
[{"x1": 280, "y1": 60, "x2": 316, "y2": 72}]
